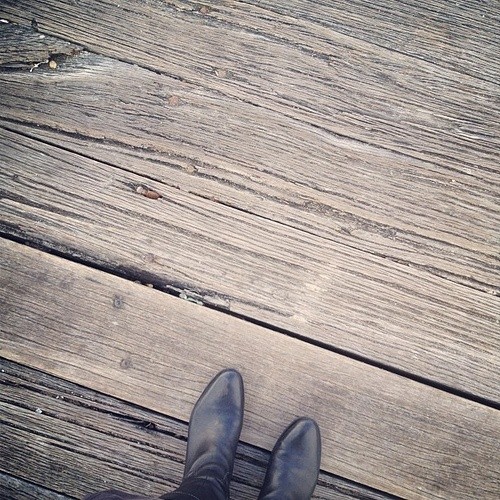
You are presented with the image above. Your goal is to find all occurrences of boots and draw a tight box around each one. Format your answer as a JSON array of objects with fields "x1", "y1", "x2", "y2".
[
  {"x1": 255, "y1": 415, "x2": 322, "y2": 500},
  {"x1": 162, "y1": 368, "x2": 244, "y2": 499}
]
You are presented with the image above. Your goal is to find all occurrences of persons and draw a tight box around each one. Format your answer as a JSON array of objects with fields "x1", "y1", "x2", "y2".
[{"x1": 77, "y1": 368, "x2": 321, "y2": 500}]
